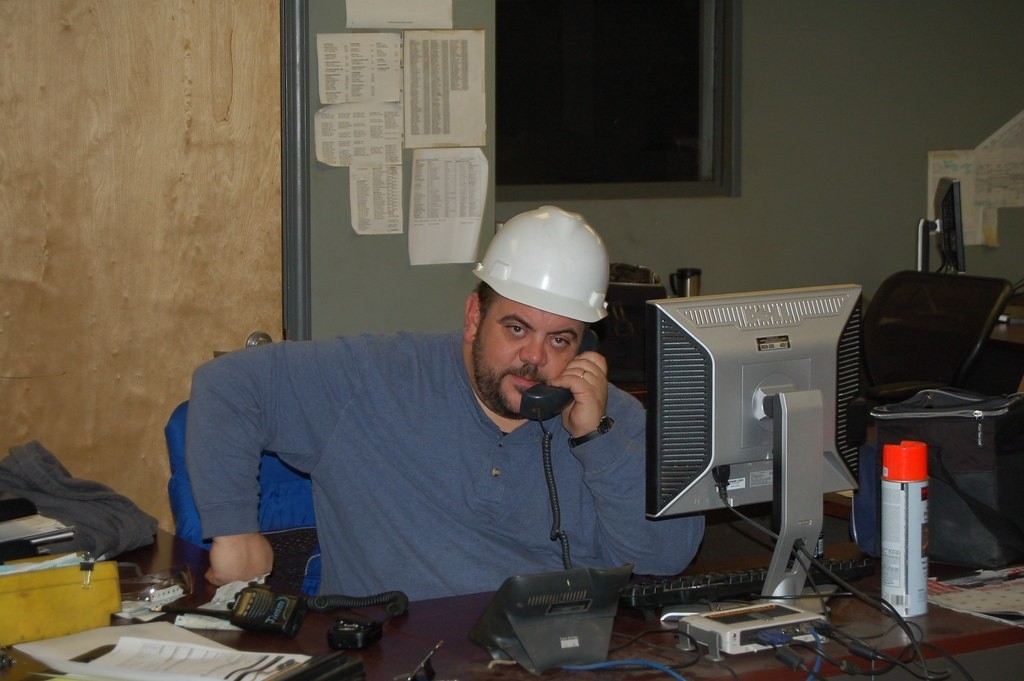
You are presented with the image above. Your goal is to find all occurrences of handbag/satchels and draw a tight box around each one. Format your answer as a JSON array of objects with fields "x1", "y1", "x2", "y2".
[{"x1": 852, "y1": 386, "x2": 1024, "y2": 566}]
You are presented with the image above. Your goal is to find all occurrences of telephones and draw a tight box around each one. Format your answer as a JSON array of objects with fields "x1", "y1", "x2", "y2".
[{"x1": 519, "y1": 330, "x2": 600, "y2": 421}]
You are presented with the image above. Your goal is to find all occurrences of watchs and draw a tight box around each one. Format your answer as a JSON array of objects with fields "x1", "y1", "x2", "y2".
[{"x1": 566, "y1": 415, "x2": 613, "y2": 449}]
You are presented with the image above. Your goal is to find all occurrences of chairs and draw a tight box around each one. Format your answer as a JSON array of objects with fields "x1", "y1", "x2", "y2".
[{"x1": 164, "y1": 399, "x2": 322, "y2": 598}]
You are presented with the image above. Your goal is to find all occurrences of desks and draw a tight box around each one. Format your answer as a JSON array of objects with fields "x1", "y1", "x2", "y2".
[{"x1": 0, "y1": 490, "x2": 1024, "y2": 681}]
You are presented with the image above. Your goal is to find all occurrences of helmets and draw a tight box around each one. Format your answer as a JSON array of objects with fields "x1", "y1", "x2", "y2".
[{"x1": 472, "y1": 206, "x2": 609, "y2": 322}]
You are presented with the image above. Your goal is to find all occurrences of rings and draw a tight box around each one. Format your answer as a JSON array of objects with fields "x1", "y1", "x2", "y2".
[{"x1": 580, "y1": 371, "x2": 586, "y2": 378}]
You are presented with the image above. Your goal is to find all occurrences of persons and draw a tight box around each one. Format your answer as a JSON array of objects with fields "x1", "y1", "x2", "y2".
[{"x1": 183, "y1": 205, "x2": 706, "y2": 602}]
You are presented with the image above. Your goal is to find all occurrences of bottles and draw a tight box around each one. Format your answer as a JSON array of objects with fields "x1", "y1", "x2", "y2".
[{"x1": 881, "y1": 440, "x2": 930, "y2": 616}]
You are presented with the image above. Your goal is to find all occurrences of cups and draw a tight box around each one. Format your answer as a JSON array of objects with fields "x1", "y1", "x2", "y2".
[{"x1": 668, "y1": 268, "x2": 701, "y2": 298}]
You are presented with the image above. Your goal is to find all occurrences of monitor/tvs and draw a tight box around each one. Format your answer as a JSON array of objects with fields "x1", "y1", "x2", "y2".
[
  {"x1": 917, "y1": 179, "x2": 966, "y2": 273},
  {"x1": 644, "y1": 282, "x2": 865, "y2": 621}
]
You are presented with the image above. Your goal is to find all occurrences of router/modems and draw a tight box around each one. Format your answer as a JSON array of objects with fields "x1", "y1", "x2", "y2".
[{"x1": 680, "y1": 602, "x2": 830, "y2": 654}]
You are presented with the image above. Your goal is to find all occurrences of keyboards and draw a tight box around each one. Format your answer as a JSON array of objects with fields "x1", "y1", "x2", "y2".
[{"x1": 617, "y1": 559, "x2": 876, "y2": 608}]
